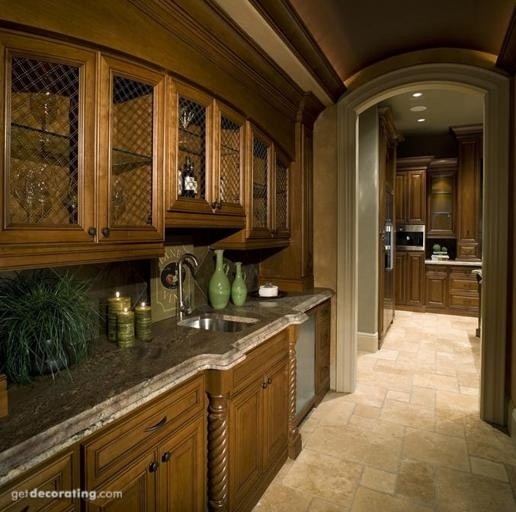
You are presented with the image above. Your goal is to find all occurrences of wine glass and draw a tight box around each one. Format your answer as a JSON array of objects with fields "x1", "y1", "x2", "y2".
[
  {"x1": 14, "y1": 161, "x2": 125, "y2": 226},
  {"x1": 178, "y1": 109, "x2": 195, "y2": 145},
  {"x1": 29, "y1": 87, "x2": 58, "y2": 144}
]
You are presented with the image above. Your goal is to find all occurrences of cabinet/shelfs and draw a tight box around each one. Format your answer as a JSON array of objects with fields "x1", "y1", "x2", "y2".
[
  {"x1": 165, "y1": 69, "x2": 246, "y2": 230},
  {"x1": 208, "y1": 117, "x2": 292, "y2": 250},
  {"x1": 229, "y1": 330, "x2": 289, "y2": 512},
  {"x1": 395, "y1": 135, "x2": 480, "y2": 317},
  {"x1": 314, "y1": 300, "x2": 331, "y2": 395},
  {"x1": 0, "y1": 19, "x2": 167, "y2": 272},
  {"x1": 0, "y1": 449, "x2": 77, "y2": 512},
  {"x1": 80, "y1": 374, "x2": 206, "y2": 512}
]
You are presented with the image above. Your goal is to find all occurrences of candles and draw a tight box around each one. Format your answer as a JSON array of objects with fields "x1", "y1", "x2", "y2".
[{"x1": 108, "y1": 292, "x2": 153, "y2": 347}]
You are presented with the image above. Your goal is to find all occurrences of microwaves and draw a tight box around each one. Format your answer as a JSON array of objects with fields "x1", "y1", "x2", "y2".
[{"x1": 395, "y1": 224, "x2": 426, "y2": 252}]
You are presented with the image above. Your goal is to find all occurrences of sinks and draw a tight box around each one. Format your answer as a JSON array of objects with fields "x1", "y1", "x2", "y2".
[{"x1": 175, "y1": 312, "x2": 261, "y2": 334}]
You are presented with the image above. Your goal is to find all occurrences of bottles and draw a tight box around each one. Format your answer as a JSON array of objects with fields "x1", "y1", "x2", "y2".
[{"x1": 177, "y1": 155, "x2": 198, "y2": 200}]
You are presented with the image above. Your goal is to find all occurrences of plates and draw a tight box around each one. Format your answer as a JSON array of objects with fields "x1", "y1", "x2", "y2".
[{"x1": 247, "y1": 288, "x2": 288, "y2": 299}]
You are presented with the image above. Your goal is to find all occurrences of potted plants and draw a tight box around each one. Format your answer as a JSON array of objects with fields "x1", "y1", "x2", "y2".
[{"x1": 0, "y1": 262, "x2": 106, "y2": 387}]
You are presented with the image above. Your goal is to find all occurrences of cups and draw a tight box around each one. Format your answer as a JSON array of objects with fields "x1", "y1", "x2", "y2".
[{"x1": 258, "y1": 285, "x2": 279, "y2": 298}]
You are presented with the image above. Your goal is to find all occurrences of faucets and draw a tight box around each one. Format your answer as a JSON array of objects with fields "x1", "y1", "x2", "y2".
[{"x1": 177, "y1": 252, "x2": 198, "y2": 318}]
